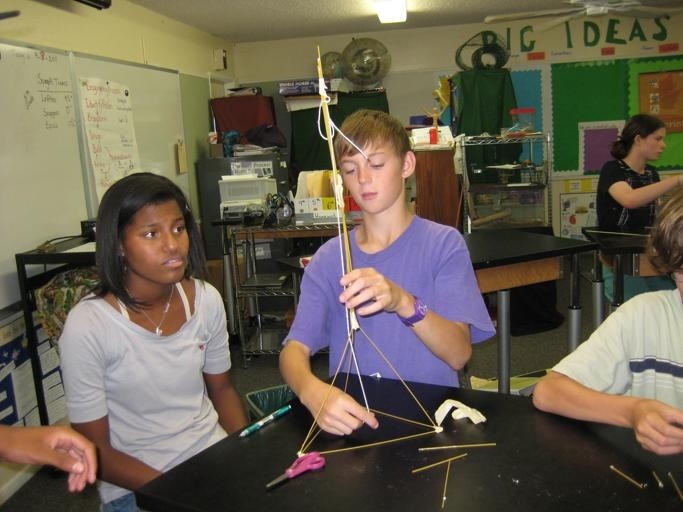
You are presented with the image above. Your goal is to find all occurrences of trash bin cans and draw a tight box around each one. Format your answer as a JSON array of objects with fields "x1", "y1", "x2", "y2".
[{"x1": 245, "y1": 384, "x2": 296, "y2": 425}]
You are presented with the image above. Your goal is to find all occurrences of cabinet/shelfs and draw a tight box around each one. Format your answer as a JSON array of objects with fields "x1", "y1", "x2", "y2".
[
  {"x1": 461, "y1": 131, "x2": 552, "y2": 234},
  {"x1": 230, "y1": 225, "x2": 359, "y2": 370}
]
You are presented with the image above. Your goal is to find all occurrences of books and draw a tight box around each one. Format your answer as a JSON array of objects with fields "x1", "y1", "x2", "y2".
[{"x1": 408, "y1": 126, "x2": 455, "y2": 151}]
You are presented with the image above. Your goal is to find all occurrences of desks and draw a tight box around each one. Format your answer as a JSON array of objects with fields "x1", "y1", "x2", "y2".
[
  {"x1": 135, "y1": 372, "x2": 683, "y2": 512},
  {"x1": 15, "y1": 235, "x2": 104, "y2": 426},
  {"x1": 464, "y1": 225, "x2": 653, "y2": 394}
]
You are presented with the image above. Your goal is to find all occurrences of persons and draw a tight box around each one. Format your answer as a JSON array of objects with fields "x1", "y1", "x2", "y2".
[
  {"x1": 596, "y1": 115, "x2": 683, "y2": 305},
  {"x1": 279, "y1": 108, "x2": 496, "y2": 436},
  {"x1": 533, "y1": 188, "x2": 683, "y2": 455},
  {"x1": 0, "y1": 425, "x2": 97, "y2": 493},
  {"x1": 58, "y1": 172, "x2": 249, "y2": 512}
]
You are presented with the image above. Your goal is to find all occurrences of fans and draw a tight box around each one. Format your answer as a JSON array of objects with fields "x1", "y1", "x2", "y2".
[{"x1": 483, "y1": 0, "x2": 683, "y2": 35}]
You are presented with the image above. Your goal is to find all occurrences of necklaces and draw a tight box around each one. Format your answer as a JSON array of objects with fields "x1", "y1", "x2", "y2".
[{"x1": 123, "y1": 284, "x2": 174, "y2": 336}]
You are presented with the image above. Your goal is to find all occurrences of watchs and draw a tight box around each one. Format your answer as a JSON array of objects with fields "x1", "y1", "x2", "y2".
[{"x1": 397, "y1": 295, "x2": 428, "y2": 327}]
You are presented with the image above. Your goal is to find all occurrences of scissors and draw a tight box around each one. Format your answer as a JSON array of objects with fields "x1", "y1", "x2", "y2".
[
  {"x1": 267, "y1": 192, "x2": 279, "y2": 203},
  {"x1": 266, "y1": 452, "x2": 326, "y2": 489}
]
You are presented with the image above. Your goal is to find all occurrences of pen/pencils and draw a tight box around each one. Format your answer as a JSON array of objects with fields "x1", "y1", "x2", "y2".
[
  {"x1": 263, "y1": 196, "x2": 280, "y2": 209},
  {"x1": 239, "y1": 404, "x2": 292, "y2": 438}
]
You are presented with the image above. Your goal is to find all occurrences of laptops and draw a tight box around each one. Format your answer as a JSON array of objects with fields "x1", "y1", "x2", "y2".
[{"x1": 240, "y1": 273, "x2": 289, "y2": 289}]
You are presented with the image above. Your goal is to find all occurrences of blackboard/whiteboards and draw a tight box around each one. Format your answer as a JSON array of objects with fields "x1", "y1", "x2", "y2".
[{"x1": 0, "y1": 37, "x2": 191, "y2": 319}]
